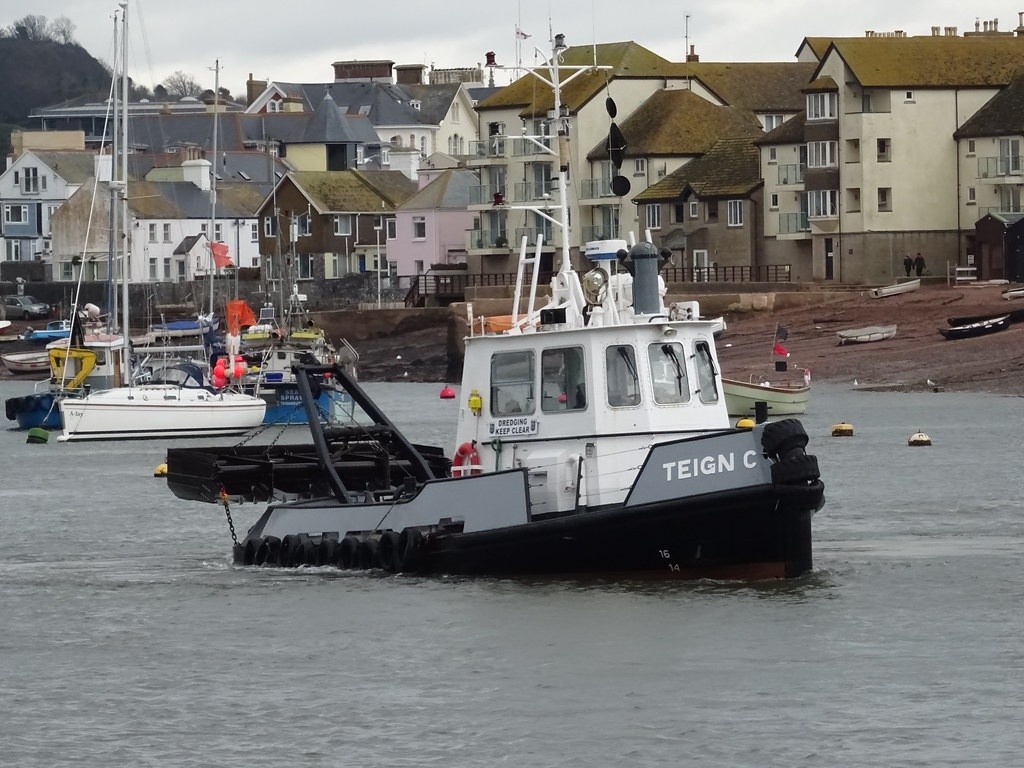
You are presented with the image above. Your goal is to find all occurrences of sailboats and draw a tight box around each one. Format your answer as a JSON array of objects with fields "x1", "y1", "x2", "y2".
[{"x1": 0, "y1": 1, "x2": 371, "y2": 444}]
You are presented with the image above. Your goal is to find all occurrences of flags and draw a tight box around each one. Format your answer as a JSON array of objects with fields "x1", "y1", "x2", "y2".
[
  {"x1": 210, "y1": 242, "x2": 234, "y2": 268},
  {"x1": 772, "y1": 323, "x2": 789, "y2": 357}
]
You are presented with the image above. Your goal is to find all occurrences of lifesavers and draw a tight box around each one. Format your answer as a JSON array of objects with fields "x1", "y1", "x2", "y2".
[{"x1": 451, "y1": 442, "x2": 479, "y2": 478}]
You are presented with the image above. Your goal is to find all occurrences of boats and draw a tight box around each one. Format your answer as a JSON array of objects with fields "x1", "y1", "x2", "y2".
[
  {"x1": 938, "y1": 313, "x2": 1012, "y2": 341},
  {"x1": 947, "y1": 308, "x2": 1023, "y2": 327},
  {"x1": 151, "y1": 51, "x2": 829, "y2": 589},
  {"x1": 720, "y1": 377, "x2": 812, "y2": 418},
  {"x1": 836, "y1": 324, "x2": 898, "y2": 343}
]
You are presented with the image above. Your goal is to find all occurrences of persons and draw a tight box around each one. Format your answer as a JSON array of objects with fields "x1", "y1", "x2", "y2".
[
  {"x1": 904, "y1": 253, "x2": 915, "y2": 277},
  {"x1": 913, "y1": 252, "x2": 926, "y2": 277},
  {"x1": 505, "y1": 399, "x2": 522, "y2": 413},
  {"x1": 572, "y1": 383, "x2": 586, "y2": 409}
]
